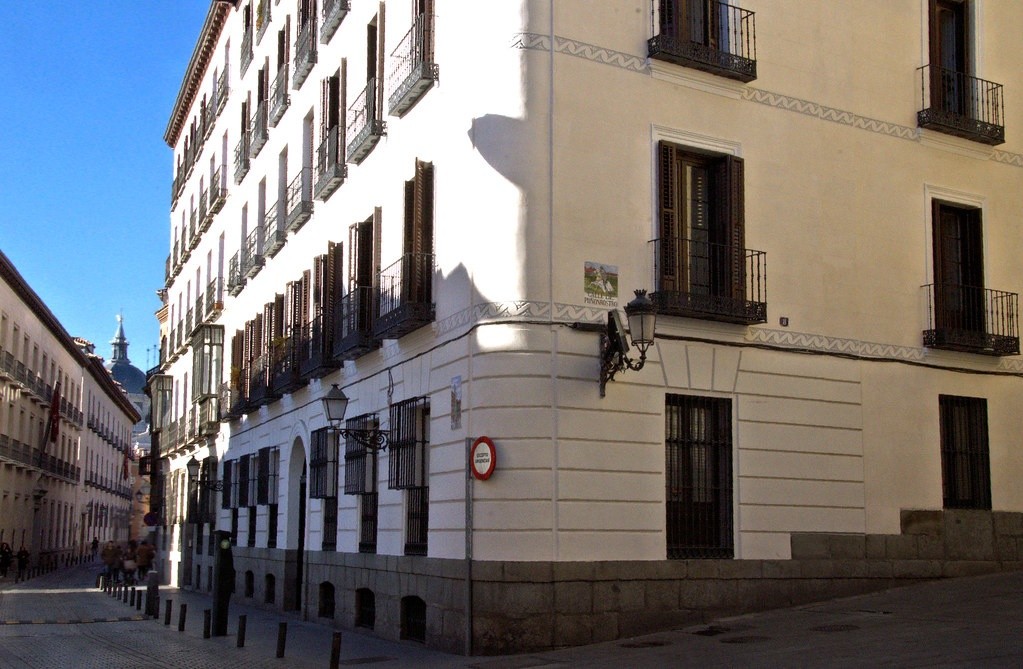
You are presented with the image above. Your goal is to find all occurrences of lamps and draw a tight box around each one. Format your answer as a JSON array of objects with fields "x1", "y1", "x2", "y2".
[
  {"x1": 321, "y1": 383, "x2": 392, "y2": 452},
  {"x1": 186, "y1": 455, "x2": 232, "y2": 494},
  {"x1": 135, "y1": 489, "x2": 166, "y2": 508},
  {"x1": 599, "y1": 288, "x2": 659, "y2": 398},
  {"x1": 603, "y1": 310, "x2": 630, "y2": 363}
]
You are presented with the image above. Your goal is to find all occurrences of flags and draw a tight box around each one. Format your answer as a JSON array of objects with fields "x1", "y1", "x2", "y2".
[
  {"x1": 51, "y1": 393, "x2": 59, "y2": 444},
  {"x1": 123, "y1": 454, "x2": 128, "y2": 480}
]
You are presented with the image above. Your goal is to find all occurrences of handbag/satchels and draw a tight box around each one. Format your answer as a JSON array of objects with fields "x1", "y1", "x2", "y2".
[
  {"x1": 124, "y1": 561, "x2": 137, "y2": 570},
  {"x1": 24, "y1": 559, "x2": 30, "y2": 564}
]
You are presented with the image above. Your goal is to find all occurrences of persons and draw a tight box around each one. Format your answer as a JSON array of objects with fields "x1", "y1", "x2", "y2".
[
  {"x1": 113, "y1": 546, "x2": 123, "y2": 582},
  {"x1": 123, "y1": 540, "x2": 137, "y2": 585},
  {"x1": 136, "y1": 541, "x2": 154, "y2": 580},
  {"x1": 0, "y1": 543, "x2": 13, "y2": 578},
  {"x1": 101, "y1": 541, "x2": 116, "y2": 582},
  {"x1": 91, "y1": 537, "x2": 99, "y2": 556},
  {"x1": 17, "y1": 547, "x2": 29, "y2": 581}
]
